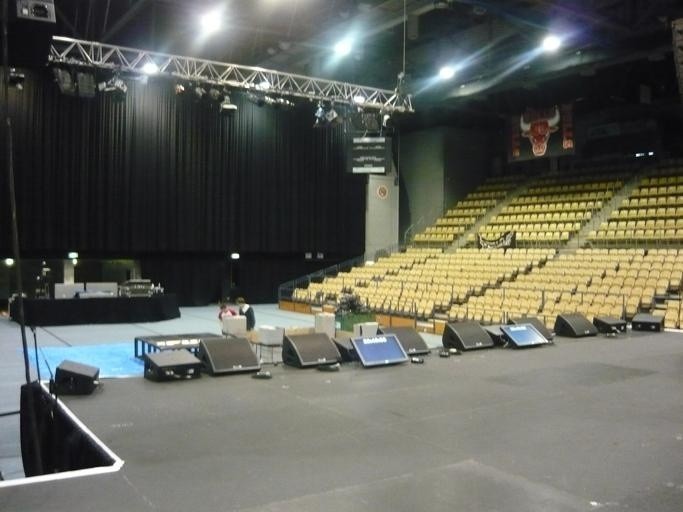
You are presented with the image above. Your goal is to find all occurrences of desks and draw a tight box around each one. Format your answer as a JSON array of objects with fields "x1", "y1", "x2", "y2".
[
  {"x1": 132, "y1": 330, "x2": 285, "y2": 364},
  {"x1": 9, "y1": 291, "x2": 180, "y2": 325}
]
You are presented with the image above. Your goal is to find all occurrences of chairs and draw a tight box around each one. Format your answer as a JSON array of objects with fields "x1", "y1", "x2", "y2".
[
  {"x1": 291, "y1": 246, "x2": 683, "y2": 333},
  {"x1": 412, "y1": 174, "x2": 683, "y2": 246}
]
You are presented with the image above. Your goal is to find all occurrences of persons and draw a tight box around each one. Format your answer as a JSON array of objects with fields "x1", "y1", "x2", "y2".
[
  {"x1": 235, "y1": 297, "x2": 255, "y2": 331},
  {"x1": 218, "y1": 305, "x2": 237, "y2": 321}
]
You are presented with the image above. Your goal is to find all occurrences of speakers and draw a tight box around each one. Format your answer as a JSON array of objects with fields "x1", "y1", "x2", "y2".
[
  {"x1": 631, "y1": 313, "x2": 664, "y2": 331},
  {"x1": 509, "y1": 317, "x2": 553, "y2": 341},
  {"x1": 378, "y1": 326, "x2": 431, "y2": 355},
  {"x1": 281, "y1": 332, "x2": 343, "y2": 367},
  {"x1": 200, "y1": 339, "x2": 261, "y2": 376},
  {"x1": 442, "y1": 320, "x2": 494, "y2": 350},
  {"x1": 553, "y1": 313, "x2": 598, "y2": 336},
  {"x1": 49, "y1": 360, "x2": 99, "y2": 396}
]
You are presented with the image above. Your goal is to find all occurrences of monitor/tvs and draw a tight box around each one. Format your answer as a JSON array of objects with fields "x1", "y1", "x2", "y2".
[
  {"x1": 499, "y1": 323, "x2": 548, "y2": 347},
  {"x1": 350, "y1": 333, "x2": 408, "y2": 368}
]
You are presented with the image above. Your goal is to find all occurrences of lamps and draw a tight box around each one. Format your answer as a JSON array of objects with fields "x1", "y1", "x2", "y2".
[{"x1": 50, "y1": 45, "x2": 414, "y2": 131}]
